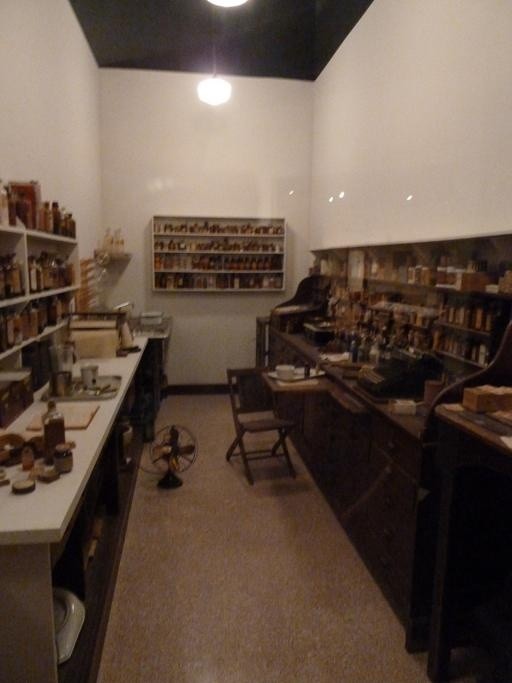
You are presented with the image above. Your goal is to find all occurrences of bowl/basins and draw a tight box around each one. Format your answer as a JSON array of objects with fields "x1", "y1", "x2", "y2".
[
  {"x1": 122, "y1": 415, "x2": 133, "y2": 447},
  {"x1": 275, "y1": 365, "x2": 296, "y2": 380}
]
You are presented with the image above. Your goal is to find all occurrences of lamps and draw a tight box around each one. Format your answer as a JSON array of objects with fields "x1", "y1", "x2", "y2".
[
  {"x1": 197, "y1": 8, "x2": 232, "y2": 106},
  {"x1": 207, "y1": 0, "x2": 246, "y2": 8}
]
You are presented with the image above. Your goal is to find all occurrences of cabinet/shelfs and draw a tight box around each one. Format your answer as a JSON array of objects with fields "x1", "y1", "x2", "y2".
[
  {"x1": 268, "y1": 329, "x2": 422, "y2": 651},
  {"x1": 0, "y1": 223, "x2": 81, "y2": 363},
  {"x1": 272, "y1": 234, "x2": 512, "y2": 436},
  {"x1": 0, "y1": 338, "x2": 157, "y2": 683},
  {"x1": 152, "y1": 213, "x2": 286, "y2": 291}
]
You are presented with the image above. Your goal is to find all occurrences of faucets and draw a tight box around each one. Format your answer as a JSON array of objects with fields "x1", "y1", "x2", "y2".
[{"x1": 112, "y1": 301, "x2": 135, "y2": 311}]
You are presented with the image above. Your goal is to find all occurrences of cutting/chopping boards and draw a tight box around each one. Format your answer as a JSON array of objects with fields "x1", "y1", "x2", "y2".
[{"x1": 29, "y1": 400, "x2": 100, "y2": 430}]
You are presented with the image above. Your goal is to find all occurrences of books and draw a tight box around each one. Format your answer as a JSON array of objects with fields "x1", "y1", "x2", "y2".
[{"x1": 25, "y1": 404, "x2": 100, "y2": 430}]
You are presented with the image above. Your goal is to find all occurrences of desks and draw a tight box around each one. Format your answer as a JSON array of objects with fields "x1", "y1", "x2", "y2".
[
  {"x1": 255, "y1": 316, "x2": 272, "y2": 364},
  {"x1": 426, "y1": 403, "x2": 512, "y2": 683}
]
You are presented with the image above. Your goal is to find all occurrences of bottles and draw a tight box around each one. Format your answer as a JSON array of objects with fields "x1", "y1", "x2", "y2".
[
  {"x1": 315, "y1": 364, "x2": 320, "y2": 374},
  {"x1": 317, "y1": 252, "x2": 491, "y2": 364},
  {"x1": 1, "y1": 181, "x2": 75, "y2": 353},
  {"x1": 154, "y1": 221, "x2": 285, "y2": 289},
  {"x1": 21, "y1": 444, "x2": 33, "y2": 468},
  {"x1": 42, "y1": 398, "x2": 66, "y2": 464},
  {"x1": 304, "y1": 361, "x2": 310, "y2": 376},
  {"x1": 46, "y1": 444, "x2": 76, "y2": 482}
]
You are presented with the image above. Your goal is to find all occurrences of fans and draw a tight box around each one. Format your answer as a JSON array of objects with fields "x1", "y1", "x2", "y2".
[{"x1": 148, "y1": 424, "x2": 198, "y2": 489}]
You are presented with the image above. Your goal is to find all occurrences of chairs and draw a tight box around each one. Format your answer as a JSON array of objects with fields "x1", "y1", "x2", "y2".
[{"x1": 226, "y1": 363, "x2": 297, "y2": 485}]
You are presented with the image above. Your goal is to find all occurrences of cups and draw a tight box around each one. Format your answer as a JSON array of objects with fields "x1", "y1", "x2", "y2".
[
  {"x1": 48, "y1": 344, "x2": 79, "y2": 396},
  {"x1": 82, "y1": 365, "x2": 99, "y2": 390}
]
